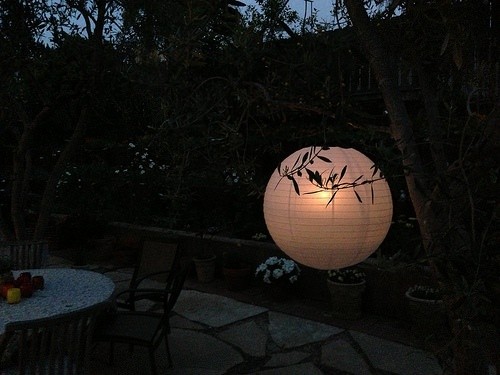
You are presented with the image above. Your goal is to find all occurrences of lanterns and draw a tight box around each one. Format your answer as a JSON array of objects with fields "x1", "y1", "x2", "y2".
[{"x1": 263, "y1": 145, "x2": 393, "y2": 271}]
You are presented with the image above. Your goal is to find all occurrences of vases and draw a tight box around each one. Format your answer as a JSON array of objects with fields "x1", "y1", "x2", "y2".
[
  {"x1": 323, "y1": 280, "x2": 366, "y2": 307},
  {"x1": 257, "y1": 276, "x2": 301, "y2": 300}
]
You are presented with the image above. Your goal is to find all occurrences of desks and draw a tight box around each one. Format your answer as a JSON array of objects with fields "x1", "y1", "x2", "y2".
[{"x1": 0, "y1": 269, "x2": 114, "y2": 375}]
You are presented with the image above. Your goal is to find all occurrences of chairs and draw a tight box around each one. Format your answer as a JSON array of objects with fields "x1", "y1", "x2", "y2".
[{"x1": 0, "y1": 237, "x2": 192, "y2": 375}]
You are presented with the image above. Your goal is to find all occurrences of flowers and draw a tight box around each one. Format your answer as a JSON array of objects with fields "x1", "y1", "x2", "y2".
[
  {"x1": 256, "y1": 255, "x2": 300, "y2": 283},
  {"x1": 329, "y1": 267, "x2": 367, "y2": 283}
]
